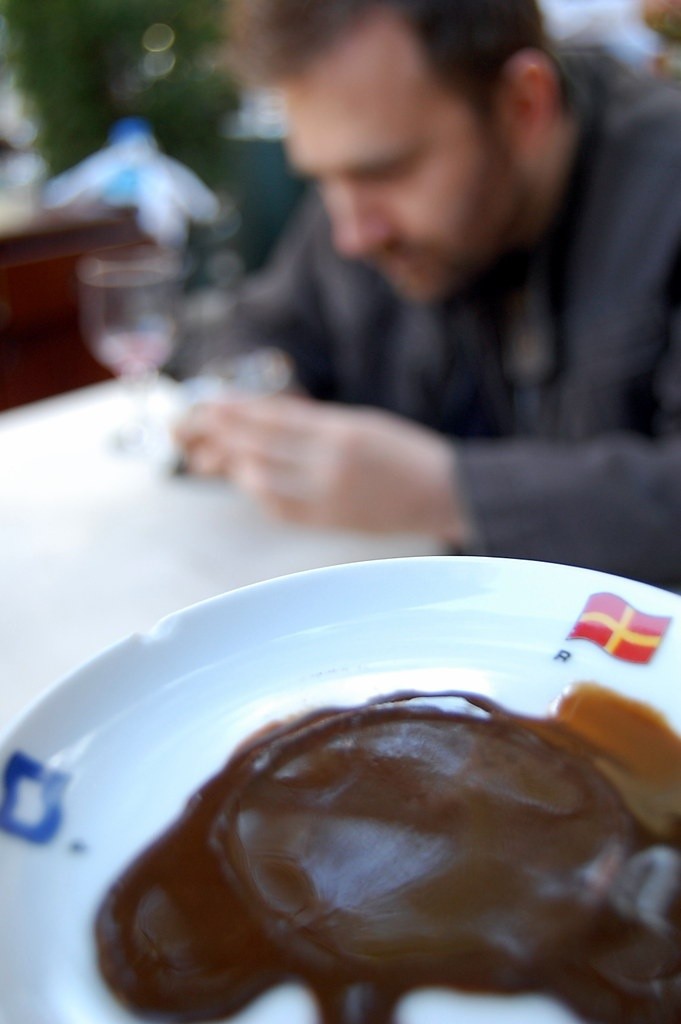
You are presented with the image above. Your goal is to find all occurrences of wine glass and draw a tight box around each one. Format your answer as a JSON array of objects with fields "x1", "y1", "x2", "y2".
[{"x1": 76, "y1": 252, "x2": 177, "y2": 448}]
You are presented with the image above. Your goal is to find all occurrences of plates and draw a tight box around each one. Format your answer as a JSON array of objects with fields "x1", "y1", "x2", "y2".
[{"x1": 0, "y1": 556, "x2": 680, "y2": 1024}]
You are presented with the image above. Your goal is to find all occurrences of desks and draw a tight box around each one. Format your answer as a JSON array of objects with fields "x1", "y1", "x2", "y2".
[{"x1": 0, "y1": 368, "x2": 450, "y2": 747}]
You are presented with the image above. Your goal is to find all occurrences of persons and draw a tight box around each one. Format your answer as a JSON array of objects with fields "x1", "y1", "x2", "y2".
[{"x1": 165, "y1": 0, "x2": 681, "y2": 593}]
